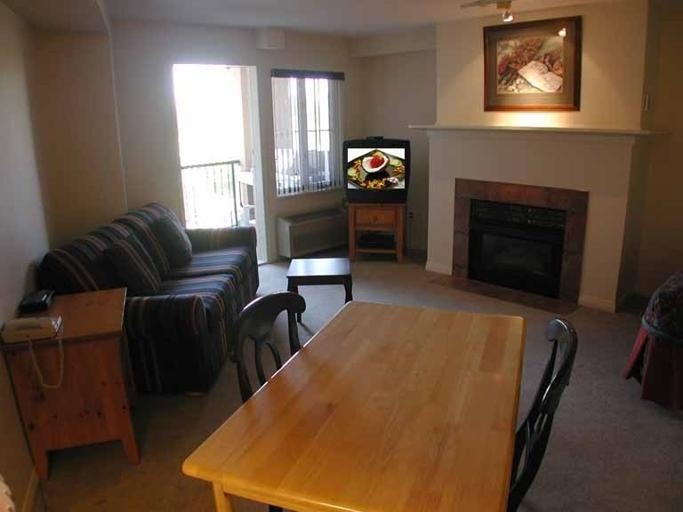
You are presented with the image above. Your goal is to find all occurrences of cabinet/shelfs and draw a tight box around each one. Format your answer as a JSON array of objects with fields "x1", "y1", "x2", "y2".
[
  {"x1": 344, "y1": 201, "x2": 407, "y2": 263},
  {"x1": 0, "y1": 287, "x2": 141, "y2": 482}
]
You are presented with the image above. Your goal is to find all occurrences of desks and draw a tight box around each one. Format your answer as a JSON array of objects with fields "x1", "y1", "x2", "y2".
[{"x1": 182, "y1": 301, "x2": 526, "y2": 512}]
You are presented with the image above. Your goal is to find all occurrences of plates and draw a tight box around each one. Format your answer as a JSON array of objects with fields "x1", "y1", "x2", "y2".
[{"x1": 345, "y1": 149, "x2": 405, "y2": 191}]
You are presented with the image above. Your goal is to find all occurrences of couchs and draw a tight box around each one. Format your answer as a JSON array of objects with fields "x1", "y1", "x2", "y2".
[{"x1": 36, "y1": 202, "x2": 259, "y2": 398}]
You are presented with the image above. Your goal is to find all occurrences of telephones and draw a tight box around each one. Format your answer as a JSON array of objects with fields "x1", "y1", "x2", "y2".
[{"x1": 1, "y1": 316, "x2": 62, "y2": 344}]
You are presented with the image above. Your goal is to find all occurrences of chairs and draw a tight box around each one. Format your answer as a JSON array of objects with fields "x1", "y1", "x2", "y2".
[
  {"x1": 229, "y1": 292, "x2": 306, "y2": 512},
  {"x1": 507, "y1": 318, "x2": 577, "y2": 512}
]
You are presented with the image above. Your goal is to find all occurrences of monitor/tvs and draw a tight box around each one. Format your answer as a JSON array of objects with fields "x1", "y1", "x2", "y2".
[{"x1": 342, "y1": 138, "x2": 412, "y2": 204}]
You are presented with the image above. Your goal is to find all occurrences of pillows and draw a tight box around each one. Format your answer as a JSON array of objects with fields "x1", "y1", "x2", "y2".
[{"x1": 99, "y1": 209, "x2": 192, "y2": 296}]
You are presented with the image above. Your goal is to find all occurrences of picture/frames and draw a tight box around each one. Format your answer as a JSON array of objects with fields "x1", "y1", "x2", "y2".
[{"x1": 483, "y1": 16, "x2": 581, "y2": 111}]
